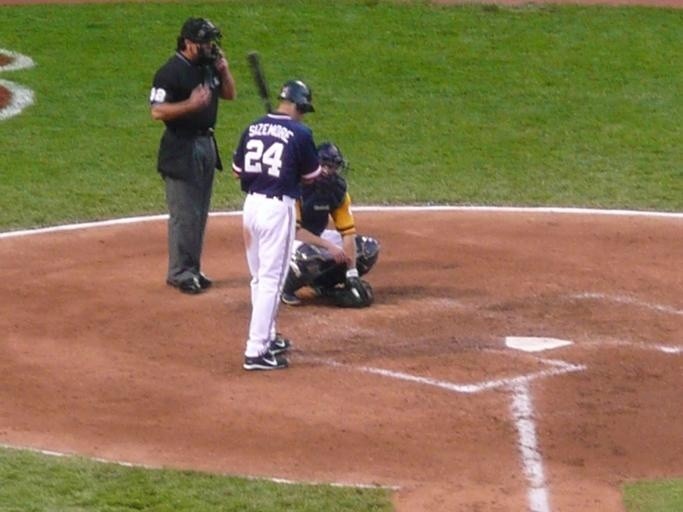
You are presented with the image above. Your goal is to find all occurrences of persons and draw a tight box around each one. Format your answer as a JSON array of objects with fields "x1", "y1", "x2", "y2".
[
  {"x1": 230, "y1": 80, "x2": 323, "y2": 370},
  {"x1": 279, "y1": 142, "x2": 380, "y2": 305},
  {"x1": 149, "y1": 17, "x2": 237, "y2": 294}
]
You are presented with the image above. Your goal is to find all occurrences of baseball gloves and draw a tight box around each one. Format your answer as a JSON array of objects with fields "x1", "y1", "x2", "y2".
[{"x1": 335, "y1": 277, "x2": 373, "y2": 309}]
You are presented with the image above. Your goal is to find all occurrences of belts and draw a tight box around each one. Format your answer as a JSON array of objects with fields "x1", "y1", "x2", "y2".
[{"x1": 248, "y1": 189, "x2": 283, "y2": 201}]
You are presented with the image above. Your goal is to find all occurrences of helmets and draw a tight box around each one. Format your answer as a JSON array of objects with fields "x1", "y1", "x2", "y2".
[
  {"x1": 181, "y1": 17, "x2": 222, "y2": 68},
  {"x1": 316, "y1": 143, "x2": 343, "y2": 168},
  {"x1": 277, "y1": 80, "x2": 316, "y2": 112}
]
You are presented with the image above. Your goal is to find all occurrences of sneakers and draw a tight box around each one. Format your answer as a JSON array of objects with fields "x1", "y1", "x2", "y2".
[
  {"x1": 267, "y1": 338, "x2": 292, "y2": 354},
  {"x1": 195, "y1": 271, "x2": 213, "y2": 288},
  {"x1": 313, "y1": 286, "x2": 343, "y2": 297},
  {"x1": 166, "y1": 278, "x2": 201, "y2": 293},
  {"x1": 243, "y1": 354, "x2": 289, "y2": 370},
  {"x1": 281, "y1": 291, "x2": 302, "y2": 305}
]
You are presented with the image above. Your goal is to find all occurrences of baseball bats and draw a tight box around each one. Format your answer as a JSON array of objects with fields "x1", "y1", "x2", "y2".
[{"x1": 246, "y1": 51, "x2": 272, "y2": 113}]
